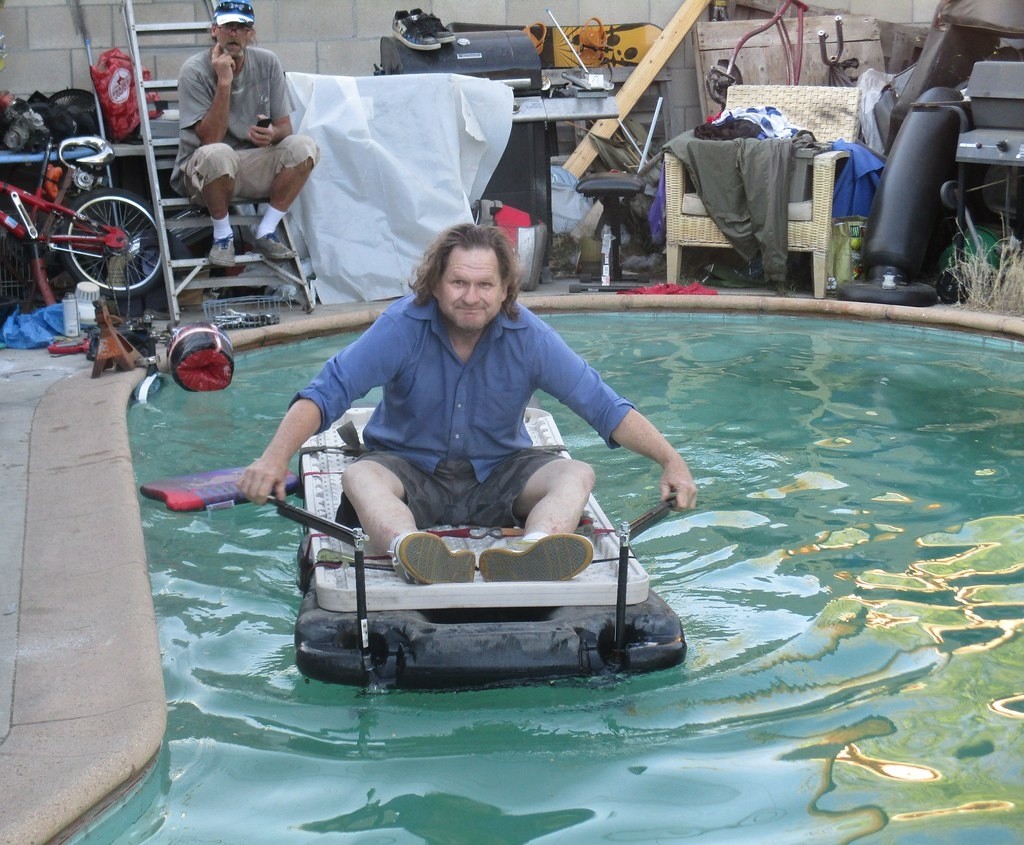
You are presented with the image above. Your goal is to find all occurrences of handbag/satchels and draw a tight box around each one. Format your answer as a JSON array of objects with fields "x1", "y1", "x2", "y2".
[
  {"x1": 826, "y1": 215, "x2": 867, "y2": 295},
  {"x1": 90, "y1": 47, "x2": 164, "y2": 144}
]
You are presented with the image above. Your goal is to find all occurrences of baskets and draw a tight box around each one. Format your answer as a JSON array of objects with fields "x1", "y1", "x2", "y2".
[{"x1": 202, "y1": 294, "x2": 281, "y2": 329}]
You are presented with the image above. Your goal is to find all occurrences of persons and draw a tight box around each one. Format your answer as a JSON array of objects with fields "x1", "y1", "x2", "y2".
[
  {"x1": 170, "y1": 0, "x2": 321, "y2": 267},
  {"x1": 235, "y1": 222, "x2": 699, "y2": 585}
]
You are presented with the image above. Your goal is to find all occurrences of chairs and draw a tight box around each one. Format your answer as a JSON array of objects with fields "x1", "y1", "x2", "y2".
[{"x1": 662, "y1": 83, "x2": 863, "y2": 300}]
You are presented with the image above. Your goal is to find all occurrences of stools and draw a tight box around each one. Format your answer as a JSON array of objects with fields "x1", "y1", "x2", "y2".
[{"x1": 568, "y1": 173, "x2": 650, "y2": 295}]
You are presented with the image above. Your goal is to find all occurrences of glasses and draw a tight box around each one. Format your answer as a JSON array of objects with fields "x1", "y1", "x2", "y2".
[
  {"x1": 216, "y1": 26, "x2": 250, "y2": 35},
  {"x1": 214, "y1": 2, "x2": 254, "y2": 16}
]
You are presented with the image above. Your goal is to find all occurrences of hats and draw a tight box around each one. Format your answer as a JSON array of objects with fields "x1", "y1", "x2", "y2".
[{"x1": 212, "y1": 0, "x2": 255, "y2": 27}]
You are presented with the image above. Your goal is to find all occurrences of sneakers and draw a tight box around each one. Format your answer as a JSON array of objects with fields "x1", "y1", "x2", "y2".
[
  {"x1": 391, "y1": 10, "x2": 441, "y2": 50},
  {"x1": 410, "y1": 8, "x2": 457, "y2": 43}
]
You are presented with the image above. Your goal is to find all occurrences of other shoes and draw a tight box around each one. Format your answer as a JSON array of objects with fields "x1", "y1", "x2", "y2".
[
  {"x1": 388, "y1": 531, "x2": 476, "y2": 584},
  {"x1": 479, "y1": 533, "x2": 594, "y2": 581},
  {"x1": 208, "y1": 236, "x2": 236, "y2": 268},
  {"x1": 253, "y1": 233, "x2": 297, "y2": 261}
]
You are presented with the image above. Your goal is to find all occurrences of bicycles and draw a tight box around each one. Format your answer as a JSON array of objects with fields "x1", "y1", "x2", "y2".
[{"x1": 0, "y1": 136, "x2": 167, "y2": 355}]
